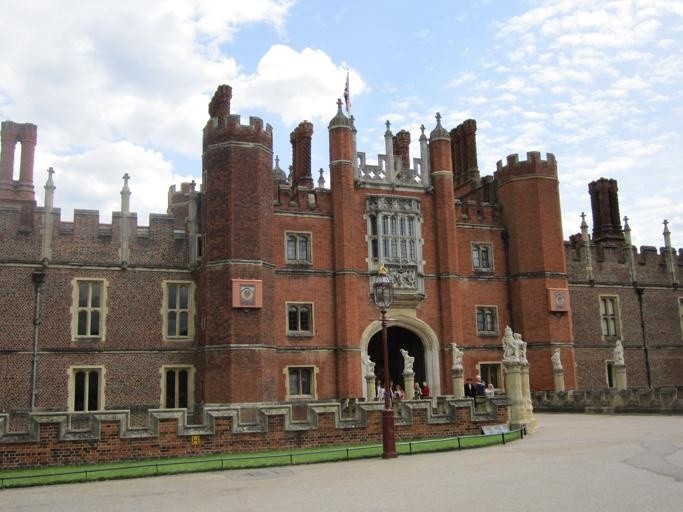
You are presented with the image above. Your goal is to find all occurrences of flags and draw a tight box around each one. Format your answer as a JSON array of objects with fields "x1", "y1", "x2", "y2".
[{"x1": 344, "y1": 72, "x2": 351, "y2": 113}]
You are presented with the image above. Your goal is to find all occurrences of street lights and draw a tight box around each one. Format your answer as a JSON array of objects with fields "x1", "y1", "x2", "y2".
[{"x1": 371, "y1": 261, "x2": 398, "y2": 459}]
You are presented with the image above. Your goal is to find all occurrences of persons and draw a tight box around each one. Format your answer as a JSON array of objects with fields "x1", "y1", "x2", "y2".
[
  {"x1": 464, "y1": 378, "x2": 476, "y2": 397},
  {"x1": 485, "y1": 384, "x2": 494, "y2": 398},
  {"x1": 376, "y1": 380, "x2": 429, "y2": 401},
  {"x1": 475, "y1": 375, "x2": 486, "y2": 396}
]
